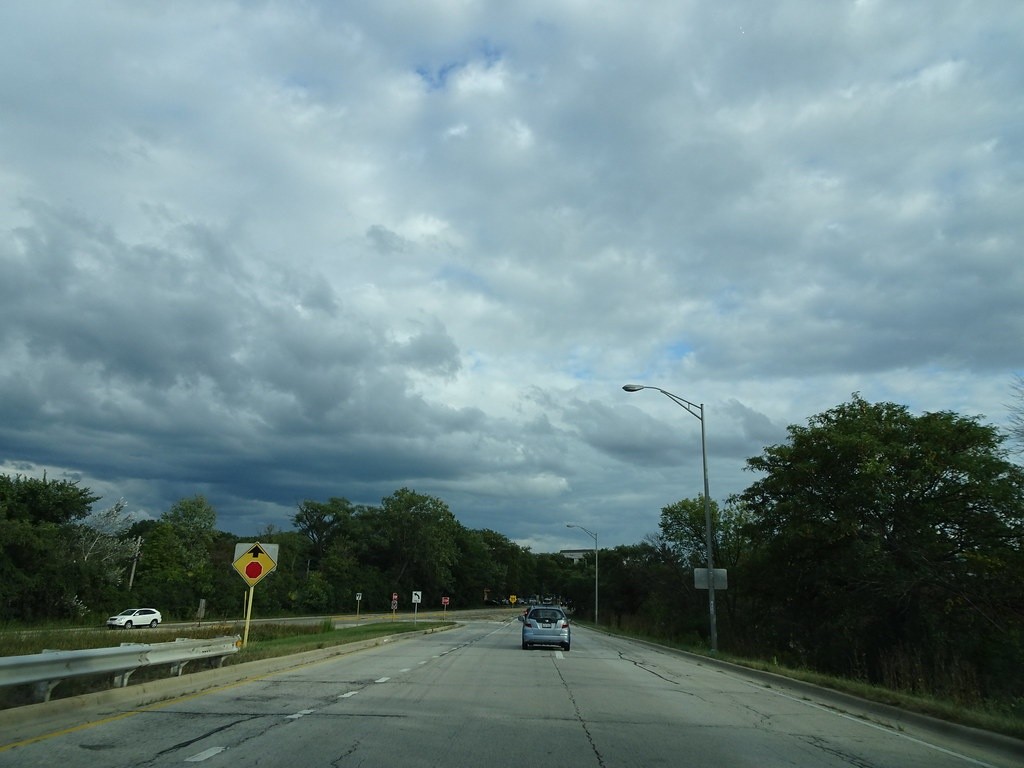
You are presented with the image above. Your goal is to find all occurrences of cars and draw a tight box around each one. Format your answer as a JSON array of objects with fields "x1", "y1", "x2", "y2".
[
  {"x1": 106, "y1": 608, "x2": 162, "y2": 630},
  {"x1": 493, "y1": 594, "x2": 565, "y2": 607}
]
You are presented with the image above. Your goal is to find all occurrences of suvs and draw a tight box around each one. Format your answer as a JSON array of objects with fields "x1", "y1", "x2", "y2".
[{"x1": 518, "y1": 604, "x2": 572, "y2": 652}]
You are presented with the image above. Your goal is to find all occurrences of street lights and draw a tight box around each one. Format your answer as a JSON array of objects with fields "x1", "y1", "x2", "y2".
[
  {"x1": 623, "y1": 384, "x2": 719, "y2": 651},
  {"x1": 566, "y1": 524, "x2": 599, "y2": 627}
]
